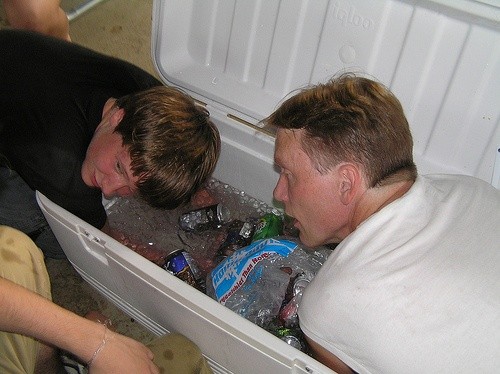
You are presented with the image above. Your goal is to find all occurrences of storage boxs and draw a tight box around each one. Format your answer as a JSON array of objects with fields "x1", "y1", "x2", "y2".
[{"x1": 35, "y1": 0, "x2": 500, "y2": 374}]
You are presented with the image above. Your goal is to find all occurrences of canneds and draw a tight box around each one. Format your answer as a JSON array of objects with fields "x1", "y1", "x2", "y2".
[
  {"x1": 213, "y1": 211, "x2": 280, "y2": 268},
  {"x1": 178, "y1": 203, "x2": 231, "y2": 234},
  {"x1": 163, "y1": 248, "x2": 206, "y2": 295},
  {"x1": 256, "y1": 268, "x2": 312, "y2": 355}
]
